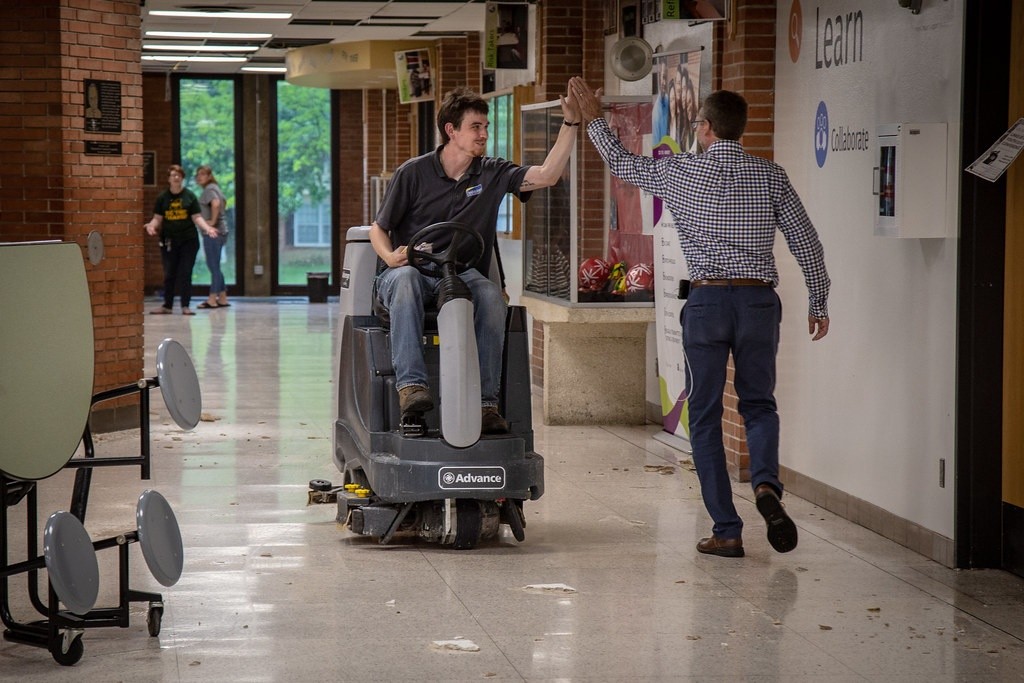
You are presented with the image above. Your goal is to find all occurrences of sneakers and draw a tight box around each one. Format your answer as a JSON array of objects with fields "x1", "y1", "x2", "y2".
[
  {"x1": 481, "y1": 405, "x2": 508, "y2": 434},
  {"x1": 180, "y1": 306, "x2": 195, "y2": 314},
  {"x1": 398, "y1": 383, "x2": 434, "y2": 412},
  {"x1": 149, "y1": 305, "x2": 172, "y2": 313}
]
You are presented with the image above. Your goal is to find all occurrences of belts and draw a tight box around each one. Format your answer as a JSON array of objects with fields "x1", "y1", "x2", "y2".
[{"x1": 690, "y1": 276, "x2": 775, "y2": 286}]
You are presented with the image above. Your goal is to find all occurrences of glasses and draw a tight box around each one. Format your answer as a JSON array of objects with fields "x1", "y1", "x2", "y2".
[{"x1": 689, "y1": 117, "x2": 703, "y2": 127}]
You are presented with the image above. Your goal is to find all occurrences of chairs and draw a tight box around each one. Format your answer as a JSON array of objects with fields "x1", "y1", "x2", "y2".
[{"x1": 369, "y1": 223, "x2": 444, "y2": 336}]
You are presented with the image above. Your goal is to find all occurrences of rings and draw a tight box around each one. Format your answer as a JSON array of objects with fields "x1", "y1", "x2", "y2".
[{"x1": 579, "y1": 93, "x2": 584, "y2": 97}]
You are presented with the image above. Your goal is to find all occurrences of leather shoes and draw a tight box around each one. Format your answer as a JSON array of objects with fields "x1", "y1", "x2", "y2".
[
  {"x1": 753, "y1": 483, "x2": 797, "y2": 553},
  {"x1": 696, "y1": 536, "x2": 744, "y2": 556}
]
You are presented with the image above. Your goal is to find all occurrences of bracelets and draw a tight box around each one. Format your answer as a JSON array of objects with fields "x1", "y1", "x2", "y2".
[{"x1": 563, "y1": 119, "x2": 581, "y2": 127}]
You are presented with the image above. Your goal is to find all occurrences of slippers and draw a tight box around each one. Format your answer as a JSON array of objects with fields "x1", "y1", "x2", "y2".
[
  {"x1": 214, "y1": 298, "x2": 231, "y2": 307},
  {"x1": 196, "y1": 301, "x2": 218, "y2": 308}
]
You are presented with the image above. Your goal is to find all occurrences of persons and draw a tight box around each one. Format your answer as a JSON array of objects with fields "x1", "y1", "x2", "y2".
[
  {"x1": 652, "y1": 59, "x2": 698, "y2": 154},
  {"x1": 364, "y1": 81, "x2": 585, "y2": 433},
  {"x1": 410, "y1": 67, "x2": 423, "y2": 98},
  {"x1": 195, "y1": 166, "x2": 233, "y2": 308},
  {"x1": 571, "y1": 75, "x2": 834, "y2": 558},
  {"x1": 143, "y1": 165, "x2": 220, "y2": 315},
  {"x1": 422, "y1": 63, "x2": 430, "y2": 96}
]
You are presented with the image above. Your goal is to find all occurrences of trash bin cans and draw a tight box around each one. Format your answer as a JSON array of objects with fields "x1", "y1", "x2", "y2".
[{"x1": 334, "y1": 226, "x2": 506, "y2": 448}]
